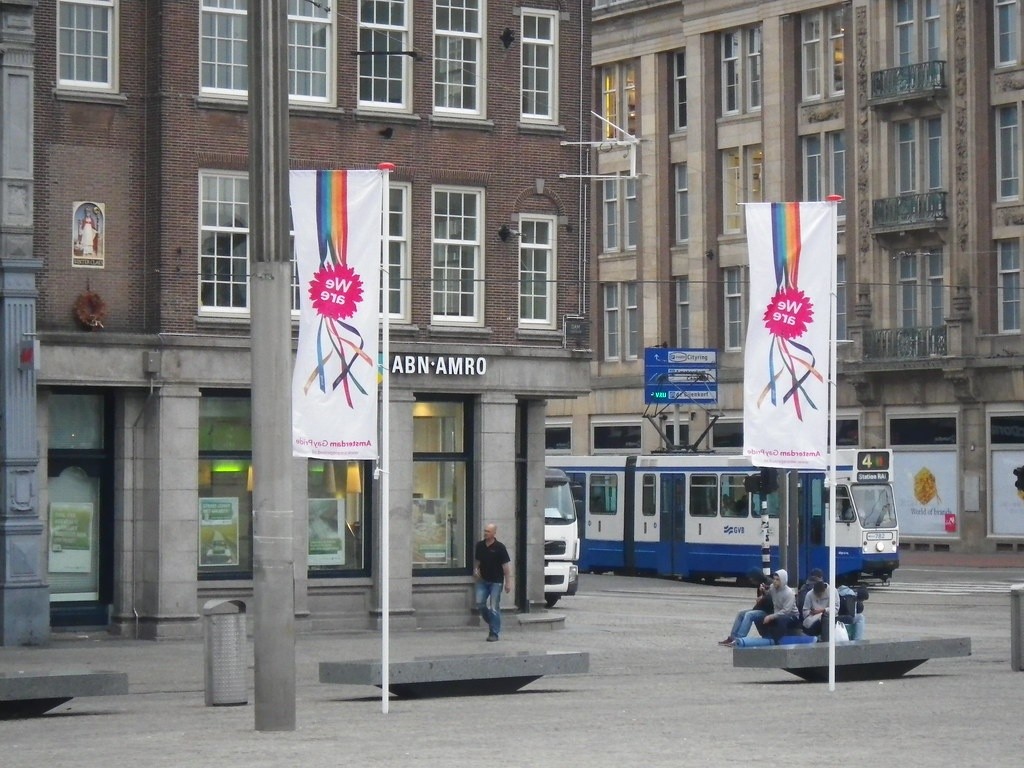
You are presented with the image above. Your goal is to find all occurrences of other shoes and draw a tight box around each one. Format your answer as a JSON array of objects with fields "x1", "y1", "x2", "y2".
[
  {"x1": 718, "y1": 637, "x2": 733, "y2": 645},
  {"x1": 487, "y1": 632, "x2": 498, "y2": 641},
  {"x1": 728, "y1": 639, "x2": 736, "y2": 647}
]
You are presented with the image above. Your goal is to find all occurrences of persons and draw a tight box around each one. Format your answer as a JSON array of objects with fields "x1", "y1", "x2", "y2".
[
  {"x1": 722, "y1": 494, "x2": 759, "y2": 517},
  {"x1": 475, "y1": 525, "x2": 512, "y2": 641},
  {"x1": 797, "y1": 569, "x2": 869, "y2": 644},
  {"x1": 845, "y1": 496, "x2": 867, "y2": 524},
  {"x1": 717, "y1": 569, "x2": 800, "y2": 646}
]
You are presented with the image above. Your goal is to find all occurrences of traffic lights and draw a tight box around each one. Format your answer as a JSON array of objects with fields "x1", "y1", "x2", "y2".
[{"x1": 1012, "y1": 466, "x2": 1024, "y2": 499}]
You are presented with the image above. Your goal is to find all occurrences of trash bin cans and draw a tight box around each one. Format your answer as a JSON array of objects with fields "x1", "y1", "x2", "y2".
[
  {"x1": 1009, "y1": 583, "x2": 1024, "y2": 673},
  {"x1": 200, "y1": 598, "x2": 250, "y2": 706}
]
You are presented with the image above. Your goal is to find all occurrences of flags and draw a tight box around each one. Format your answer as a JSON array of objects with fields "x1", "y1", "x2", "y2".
[
  {"x1": 744, "y1": 202, "x2": 834, "y2": 469},
  {"x1": 289, "y1": 169, "x2": 383, "y2": 460}
]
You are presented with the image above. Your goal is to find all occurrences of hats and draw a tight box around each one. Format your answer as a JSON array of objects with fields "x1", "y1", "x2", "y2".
[
  {"x1": 814, "y1": 581, "x2": 825, "y2": 592},
  {"x1": 810, "y1": 568, "x2": 823, "y2": 576}
]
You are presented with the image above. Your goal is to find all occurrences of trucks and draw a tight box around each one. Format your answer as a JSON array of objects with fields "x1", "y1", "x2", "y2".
[{"x1": 544, "y1": 468, "x2": 585, "y2": 610}]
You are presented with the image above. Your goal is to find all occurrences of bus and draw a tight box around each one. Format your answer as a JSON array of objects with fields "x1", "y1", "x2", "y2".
[{"x1": 545, "y1": 374, "x2": 901, "y2": 591}]
